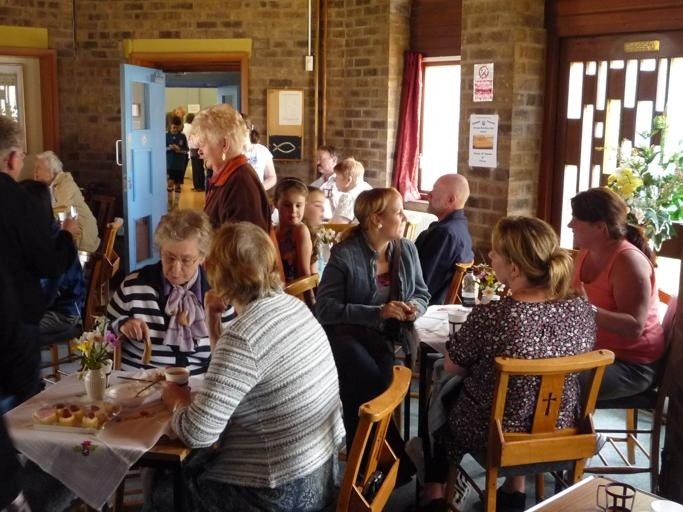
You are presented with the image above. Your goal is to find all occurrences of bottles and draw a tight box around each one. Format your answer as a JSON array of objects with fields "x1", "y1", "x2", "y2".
[{"x1": 462, "y1": 267, "x2": 480, "y2": 312}]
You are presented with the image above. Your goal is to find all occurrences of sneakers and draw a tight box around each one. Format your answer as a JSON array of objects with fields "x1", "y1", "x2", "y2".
[
  {"x1": 480, "y1": 490, "x2": 526, "y2": 512},
  {"x1": 562, "y1": 431, "x2": 608, "y2": 482}
]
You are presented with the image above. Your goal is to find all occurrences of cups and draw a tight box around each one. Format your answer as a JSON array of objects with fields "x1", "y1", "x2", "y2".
[
  {"x1": 448, "y1": 311, "x2": 471, "y2": 344},
  {"x1": 164, "y1": 367, "x2": 190, "y2": 387}
]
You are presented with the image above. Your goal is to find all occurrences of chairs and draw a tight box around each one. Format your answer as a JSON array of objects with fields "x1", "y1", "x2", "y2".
[
  {"x1": 570, "y1": 294, "x2": 680, "y2": 491},
  {"x1": 443, "y1": 259, "x2": 475, "y2": 303},
  {"x1": 334, "y1": 366, "x2": 415, "y2": 511},
  {"x1": 88, "y1": 194, "x2": 116, "y2": 232},
  {"x1": 284, "y1": 272, "x2": 323, "y2": 309},
  {"x1": 40, "y1": 217, "x2": 124, "y2": 382},
  {"x1": 483, "y1": 350, "x2": 613, "y2": 512}
]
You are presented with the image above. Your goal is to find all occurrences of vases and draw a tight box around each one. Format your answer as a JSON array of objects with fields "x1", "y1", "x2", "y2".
[
  {"x1": 84, "y1": 369, "x2": 107, "y2": 402},
  {"x1": 481, "y1": 295, "x2": 500, "y2": 304},
  {"x1": 320, "y1": 243, "x2": 334, "y2": 281}
]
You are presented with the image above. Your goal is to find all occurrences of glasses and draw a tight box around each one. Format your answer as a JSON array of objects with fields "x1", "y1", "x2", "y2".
[{"x1": 164, "y1": 255, "x2": 200, "y2": 266}]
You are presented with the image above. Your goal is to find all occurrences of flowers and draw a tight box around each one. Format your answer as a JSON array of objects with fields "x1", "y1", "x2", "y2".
[
  {"x1": 317, "y1": 227, "x2": 334, "y2": 243},
  {"x1": 474, "y1": 262, "x2": 504, "y2": 298},
  {"x1": 604, "y1": 114, "x2": 683, "y2": 259},
  {"x1": 70, "y1": 315, "x2": 122, "y2": 379}
]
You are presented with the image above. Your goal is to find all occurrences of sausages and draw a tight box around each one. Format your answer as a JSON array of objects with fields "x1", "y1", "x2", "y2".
[{"x1": 141, "y1": 333, "x2": 152, "y2": 365}]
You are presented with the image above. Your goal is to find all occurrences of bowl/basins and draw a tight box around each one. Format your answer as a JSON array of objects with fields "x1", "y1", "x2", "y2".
[{"x1": 103, "y1": 382, "x2": 156, "y2": 407}]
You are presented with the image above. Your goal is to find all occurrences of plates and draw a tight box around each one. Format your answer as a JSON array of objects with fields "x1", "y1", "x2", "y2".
[{"x1": 32, "y1": 407, "x2": 121, "y2": 433}]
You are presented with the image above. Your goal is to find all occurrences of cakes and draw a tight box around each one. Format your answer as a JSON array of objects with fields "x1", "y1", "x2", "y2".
[
  {"x1": 59, "y1": 410, "x2": 75, "y2": 427},
  {"x1": 49, "y1": 402, "x2": 67, "y2": 415},
  {"x1": 81, "y1": 412, "x2": 98, "y2": 430},
  {"x1": 32, "y1": 408, "x2": 59, "y2": 426},
  {"x1": 87, "y1": 405, "x2": 106, "y2": 423},
  {"x1": 69, "y1": 404, "x2": 84, "y2": 422}
]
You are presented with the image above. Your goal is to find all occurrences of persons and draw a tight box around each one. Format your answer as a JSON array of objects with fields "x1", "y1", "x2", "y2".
[
  {"x1": 38, "y1": 230, "x2": 84, "y2": 334},
  {"x1": 310, "y1": 145, "x2": 336, "y2": 220},
  {"x1": 328, "y1": 160, "x2": 374, "y2": 223},
  {"x1": 240, "y1": 113, "x2": 277, "y2": 192},
  {"x1": 192, "y1": 104, "x2": 285, "y2": 286},
  {"x1": 182, "y1": 113, "x2": 194, "y2": 141},
  {"x1": 568, "y1": 187, "x2": 666, "y2": 400},
  {"x1": 272, "y1": 177, "x2": 317, "y2": 308},
  {"x1": 166, "y1": 105, "x2": 187, "y2": 131},
  {"x1": 413, "y1": 216, "x2": 598, "y2": 512},
  {"x1": 17, "y1": 179, "x2": 59, "y2": 240},
  {"x1": 189, "y1": 135, "x2": 205, "y2": 192},
  {"x1": 413, "y1": 174, "x2": 474, "y2": 305},
  {"x1": 166, "y1": 116, "x2": 189, "y2": 213},
  {"x1": 1, "y1": 115, "x2": 46, "y2": 409},
  {"x1": 36, "y1": 151, "x2": 102, "y2": 268},
  {"x1": 164, "y1": 223, "x2": 346, "y2": 512},
  {"x1": 101, "y1": 210, "x2": 236, "y2": 512},
  {"x1": 303, "y1": 185, "x2": 326, "y2": 285},
  {"x1": 315, "y1": 187, "x2": 431, "y2": 476}
]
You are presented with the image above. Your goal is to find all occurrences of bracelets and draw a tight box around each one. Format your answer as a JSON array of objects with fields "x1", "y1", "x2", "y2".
[{"x1": 172, "y1": 398, "x2": 188, "y2": 413}]
